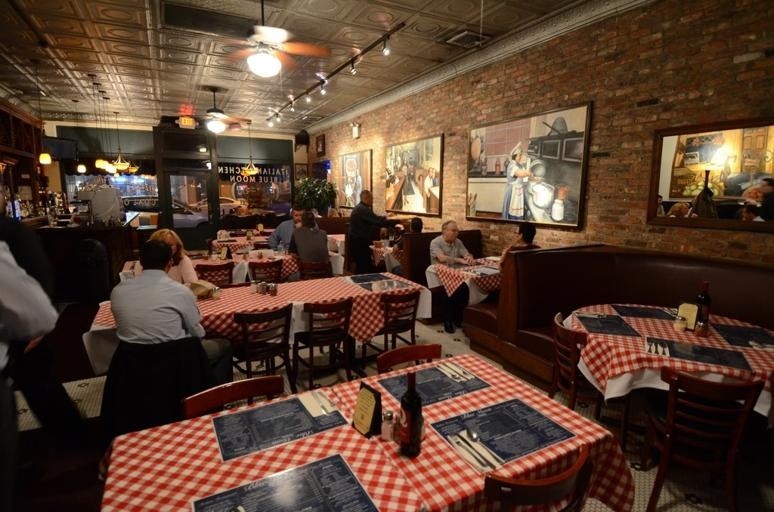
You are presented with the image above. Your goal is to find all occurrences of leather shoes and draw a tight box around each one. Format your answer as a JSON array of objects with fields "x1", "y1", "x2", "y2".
[{"x1": 444, "y1": 319, "x2": 454, "y2": 333}]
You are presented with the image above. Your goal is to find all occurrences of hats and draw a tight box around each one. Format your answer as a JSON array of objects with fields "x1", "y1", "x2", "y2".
[{"x1": 510, "y1": 141, "x2": 522, "y2": 155}]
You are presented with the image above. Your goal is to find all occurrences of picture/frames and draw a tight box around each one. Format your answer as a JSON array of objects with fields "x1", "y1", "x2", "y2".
[
  {"x1": 645, "y1": 118, "x2": 773, "y2": 233},
  {"x1": 383, "y1": 135, "x2": 444, "y2": 219},
  {"x1": 341, "y1": 149, "x2": 374, "y2": 209},
  {"x1": 314, "y1": 134, "x2": 325, "y2": 157},
  {"x1": 465, "y1": 102, "x2": 592, "y2": 226},
  {"x1": 295, "y1": 163, "x2": 307, "y2": 178}
]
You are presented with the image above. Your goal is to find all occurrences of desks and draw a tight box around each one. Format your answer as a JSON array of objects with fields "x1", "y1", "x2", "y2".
[{"x1": 31, "y1": 198, "x2": 139, "y2": 298}]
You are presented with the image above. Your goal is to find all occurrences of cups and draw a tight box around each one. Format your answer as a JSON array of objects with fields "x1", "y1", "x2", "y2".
[{"x1": 213, "y1": 286, "x2": 222, "y2": 298}]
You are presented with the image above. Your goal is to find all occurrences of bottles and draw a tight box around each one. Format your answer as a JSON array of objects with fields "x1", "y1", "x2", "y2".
[
  {"x1": 257, "y1": 251, "x2": 263, "y2": 259},
  {"x1": 481, "y1": 162, "x2": 488, "y2": 176},
  {"x1": 694, "y1": 320, "x2": 709, "y2": 338},
  {"x1": 250, "y1": 279, "x2": 257, "y2": 292},
  {"x1": 246, "y1": 230, "x2": 252, "y2": 240},
  {"x1": 277, "y1": 240, "x2": 284, "y2": 255},
  {"x1": 558, "y1": 184, "x2": 568, "y2": 207},
  {"x1": 380, "y1": 409, "x2": 394, "y2": 441},
  {"x1": 551, "y1": 199, "x2": 565, "y2": 220},
  {"x1": 398, "y1": 370, "x2": 423, "y2": 459},
  {"x1": 673, "y1": 315, "x2": 688, "y2": 332},
  {"x1": 211, "y1": 251, "x2": 218, "y2": 260},
  {"x1": 494, "y1": 157, "x2": 502, "y2": 175},
  {"x1": 261, "y1": 281, "x2": 267, "y2": 295},
  {"x1": 696, "y1": 279, "x2": 712, "y2": 325}
]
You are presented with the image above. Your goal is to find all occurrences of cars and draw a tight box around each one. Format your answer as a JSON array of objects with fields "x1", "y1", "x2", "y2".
[{"x1": 189, "y1": 197, "x2": 249, "y2": 217}]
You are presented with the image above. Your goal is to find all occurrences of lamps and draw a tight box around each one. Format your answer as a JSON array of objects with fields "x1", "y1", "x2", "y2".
[
  {"x1": 382, "y1": 40, "x2": 389, "y2": 56},
  {"x1": 108, "y1": 112, "x2": 130, "y2": 171},
  {"x1": 206, "y1": 122, "x2": 225, "y2": 135},
  {"x1": 93, "y1": 82, "x2": 108, "y2": 171},
  {"x1": 102, "y1": 97, "x2": 116, "y2": 173},
  {"x1": 126, "y1": 161, "x2": 140, "y2": 177},
  {"x1": 350, "y1": 61, "x2": 357, "y2": 78},
  {"x1": 319, "y1": 81, "x2": 325, "y2": 97},
  {"x1": 239, "y1": 121, "x2": 258, "y2": 177},
  {"x1": 87, "y1": 74, "x2": 104, "y2": 169},
  {"x1": 248, "y1": 52, "x2": 281, "y2": 78},
  {"x1": 99, "y1": 91, "x2": 113, "y2": 172},
  {"x1": 30, "y1": 58, "x2": 52, "y2": 168},
  {"x1": 347, "y1": 121, "x2": 361, "y2": 140},
  {"x1": 71, "y1": 98, "x2": 86, "y2": 174}
]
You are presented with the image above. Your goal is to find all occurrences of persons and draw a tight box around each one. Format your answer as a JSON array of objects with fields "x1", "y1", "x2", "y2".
[
  {"x1": 391, "y1": 217, "x2": 423, "y2": 257},
  {"x1": 657, "y1": 194, "x2": 665, "y2": 217},
  {"x1": 424, "y1": 167, "x2": 439, "y2": 199},
  {"x1": 502, "y1": 142, "x2": 530, "y2": 221},
  {"x1": 147, "y1": 229, "x2": 198, "y2": 285},
  {"x1": 0, "y1": 240, "x2": 60, "y2": 450},
  {"x1": 745, "y1": 177, "x2": 774, "y2": 221},
  {"x1": 287, "y1": 211, "x2": 330, "y2": 282},
  {"x1": 347, "y1": 190, "x2": 389, "y2": 273},
  {"x1": 429, "y1": 220, "x2": 473, "y2": 333},
  {"x1": 268, "y1": 205, "x2": 319, "y2": 250},
  {"x1": 110, "y1": 238, "x2": 233, "y2": 382},
  {"x1": 499, "y1": 221, "x2": 541, "y2": 269}
]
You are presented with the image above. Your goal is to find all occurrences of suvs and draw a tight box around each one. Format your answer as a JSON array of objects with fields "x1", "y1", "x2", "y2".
[{"x1": 121, "y1": 195, "x2": 209, "y2": 237}]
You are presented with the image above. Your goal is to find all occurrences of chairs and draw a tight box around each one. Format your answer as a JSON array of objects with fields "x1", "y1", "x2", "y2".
[
  {"x1": 136, "y1": 211, "x2": 165, "y2": 251},
  {"x1": 291, "y1": 297, "x2": 359, "y2": 389},
  {"x1": 297, "y1": 258, "x2": 332, "y2": 277},
  {"x1": 549, "y1": 312, "x2": 629, "y2": 448},
  {"x1": 231, "y1": 302, "x2": 300, "y2": 404},
  {"x1": 372, "y1": 340, "x2": 442, "y2": 374},
  {"x1": 248, "y1": 259, "x2": 283, "y2": 282},
  {"x1": 361, "y1": 292, "x2": 425, "y2": 367},
  {"x1": 247, "y1": 241, "x2": 272, "y2": 248},
  {"x1": 634, "y1": 362, "x2": 765, "y2": 512},
  {"x1": 481, "y1": 444, "x2": 589, "y2": 512},
  {"x1": 182, "y1": 374, "x2": 287, "y2": 416},
  {"x1": 194, "y1": 262, "x2": 234, "y2": 285}
]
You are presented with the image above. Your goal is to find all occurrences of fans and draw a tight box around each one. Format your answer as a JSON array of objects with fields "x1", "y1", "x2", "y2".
[
  {"x1": 206, "y1": 1, "x2": 330, "y2": 85},
  {"x1": 176, "y1": 85, "x2": 253, "y2": 133}
]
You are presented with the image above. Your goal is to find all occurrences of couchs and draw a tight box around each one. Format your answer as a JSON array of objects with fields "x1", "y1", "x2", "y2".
[
  {"x1": 462, "y1": 242, "x2": 510, "y2": 365},
  {"x1": 311, "y1": 218, "x2": 424, "y2": 256},
  {"x1": 403, "y1": 229, "x2": 482, "y2": 325},
  {"x1": 507, "y1": 244, "x2": 774, "y2": 393}
]
